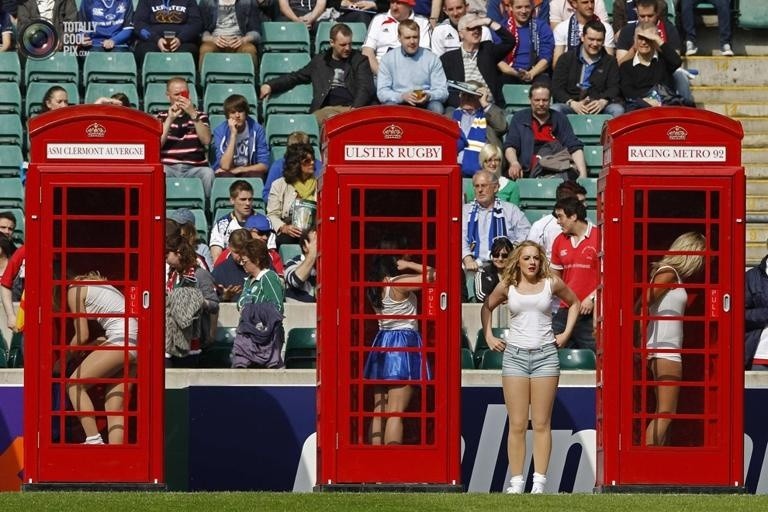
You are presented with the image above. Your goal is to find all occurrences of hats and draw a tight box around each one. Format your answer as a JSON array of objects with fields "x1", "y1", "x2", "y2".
[
  {"x1": 167, "y1": 208, "x2": 196, "y2": 225},
  {"x1": 246, "y1": 213, "x2": 276, "y2": 234}
]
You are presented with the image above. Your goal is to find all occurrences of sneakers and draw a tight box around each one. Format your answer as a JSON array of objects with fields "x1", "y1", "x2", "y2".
[
  {"x1": 530, "y1": 477, "x2": 547, "y2": 494},
  {"x1": 506, "y1": 479, "x2": 526, "y2": 494}
]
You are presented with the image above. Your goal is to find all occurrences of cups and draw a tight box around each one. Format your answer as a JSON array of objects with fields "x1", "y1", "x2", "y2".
[{"x1": 163, "y1": 30, "x2": 176, "y2": 52}]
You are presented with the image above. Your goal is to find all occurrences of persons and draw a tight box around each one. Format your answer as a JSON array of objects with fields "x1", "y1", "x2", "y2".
[
  {"x1": 480, "y1": 240, "x2": 582, "y2": 495},
  {"x1": 363, "y1": 226, "x2": 436, "y2": 445},
  {"x1": 1, "y1": 0, "x2": 768, "y2": 374},
  {"x1": 53, "y1": 253, "x2": 139, "y2": 445},
  {"x1": 635, "y1": 231, "x2": 715, "y2": 446}
]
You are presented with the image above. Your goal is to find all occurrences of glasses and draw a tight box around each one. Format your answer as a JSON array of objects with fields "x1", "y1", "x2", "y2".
[
  {"x1": 461, "y1": 27, "x2": 482, "y2": 32},
  {"x1": 300, "y1": 156, "x2": 316, "y2": 166},
  {"x1": 249, "y1": 229, "x2": 272, "y2": 237},
  {"x1": 491, "y1": 252, "x2": 508, "y2": 258}
]
